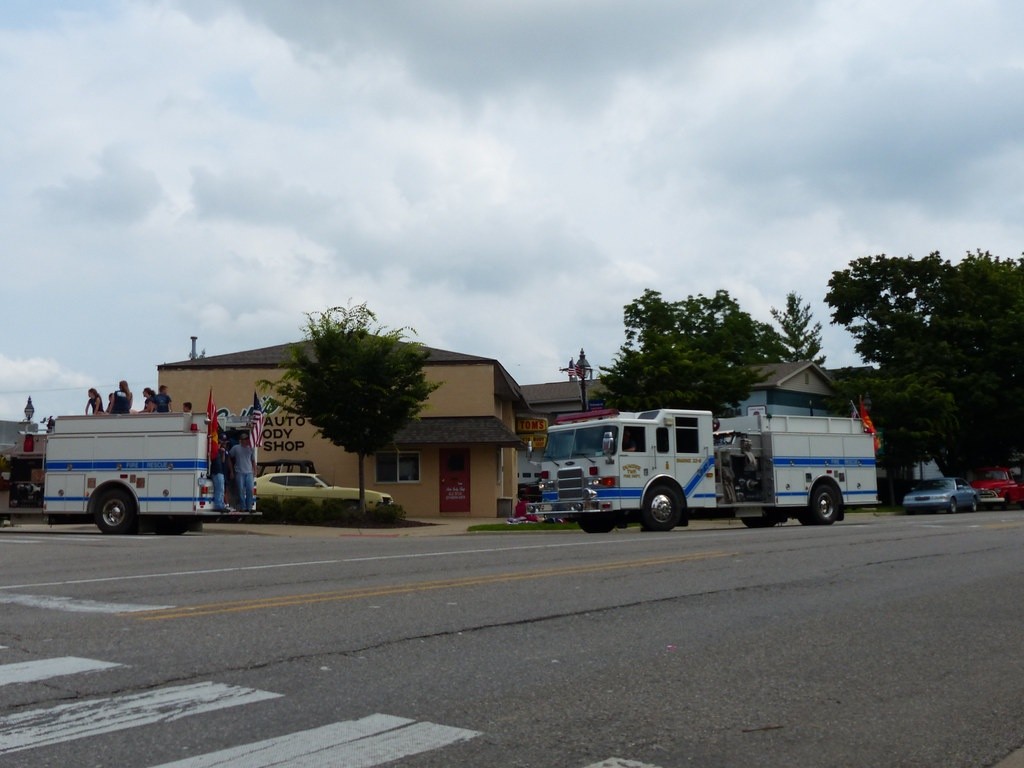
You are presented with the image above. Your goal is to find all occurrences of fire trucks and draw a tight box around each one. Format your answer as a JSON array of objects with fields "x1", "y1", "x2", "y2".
[
  {"x1": 524, "y1": 408, "x2": 881, "y2": 535},
  {"x1": 0, "y1": 409, "x2": 262, "y2": 537}
]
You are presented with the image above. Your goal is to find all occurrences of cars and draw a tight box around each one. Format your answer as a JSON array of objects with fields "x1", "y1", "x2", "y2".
[
  {"x1": 902, "y1": 476, "x2": 981, "y2": 513},
  {"x1": 254, "y1": 472, "x2": 392, "y2": 517},
  {"x1": 970, "y1": 466, "x2": 1024, "y2": 509}
]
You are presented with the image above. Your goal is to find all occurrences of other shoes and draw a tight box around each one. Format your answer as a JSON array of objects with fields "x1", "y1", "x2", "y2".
[
  {"x1": 239, "y1": 508, "x2": 256, "y2": 513},
  {"x1": 214, "y1": 508, "x2": 230, "y2": 513}
]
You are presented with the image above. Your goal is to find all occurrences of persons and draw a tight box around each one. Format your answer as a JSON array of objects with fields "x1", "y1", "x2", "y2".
[
  {"x1": 153, "y1": 386, "x2": 173, "y2": 413},
  {"x1": 211, "y1": 432, "x2": 230, "y2": 513},
  {"x1": 228, "y1": 434, "x2": 257, "y2": 512},
  {"x1": 105, "y1": 393, "x2": 114, "y2": 414},
  {"x1": 85, "y1": 387, "x2": 103, "y2": 415},
  {"x1": 110, "y1": 380, "x2": 132, "y2": 413},
  {"x1": 183, "y1": 402, "x2": 192, "y2": 413},
  {"x1": 138, "y1": 388, "x2": 156, "y2": 413},
  {"x1": 623, "y1": 431, "x2": 635, "y2": 452}
]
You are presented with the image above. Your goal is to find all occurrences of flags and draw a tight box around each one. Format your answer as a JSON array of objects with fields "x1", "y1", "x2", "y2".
[
  {"x1": 207, "y1": 386, "x2": 219, "y2": 460},
  {"x1": 250, "y1": 389, "x2": 262, "y2": 448},
  {"x1": 848, "y1": 400, "x2": 857, "y2": 417},
  {"x1": 860, "y1": 402, "x2": 878, "y2": 449},
  {"x1": 569, "y1": 360, "x2": 582, "y2": 377}
]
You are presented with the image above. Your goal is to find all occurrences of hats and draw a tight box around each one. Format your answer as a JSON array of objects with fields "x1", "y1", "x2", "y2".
[
  {"x1": 239, "y1": 433, "x2": 248, "y2": 439},
  {"x1": 218, "y1": 434, "x2": 231, "y2": 443}
]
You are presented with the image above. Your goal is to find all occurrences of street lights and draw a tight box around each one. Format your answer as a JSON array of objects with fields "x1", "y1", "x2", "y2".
[{"x1": 574, "y1": 348, "x2": 594, "y2": 409}]
